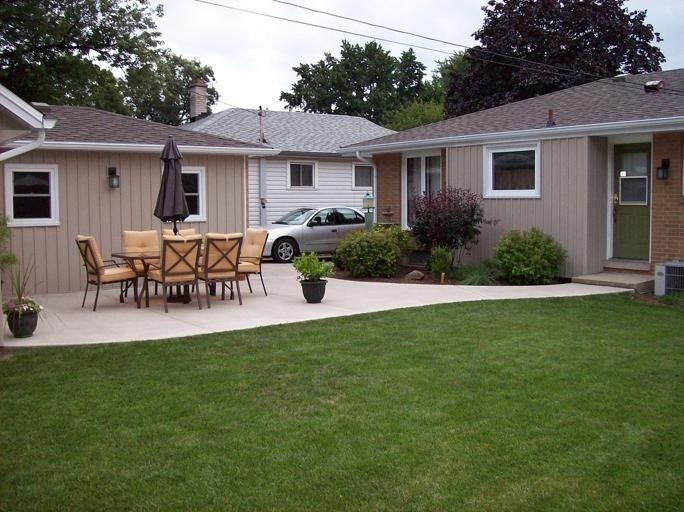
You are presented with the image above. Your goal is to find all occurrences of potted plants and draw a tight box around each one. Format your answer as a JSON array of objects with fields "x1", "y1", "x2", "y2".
[{"x1": 291, "y1": 251, "x2": 335, "y2": 302}]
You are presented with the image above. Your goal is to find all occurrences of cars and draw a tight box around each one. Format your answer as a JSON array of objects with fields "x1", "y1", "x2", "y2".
[
  {"x1": 263, "y1": 205, "x2": 366, "y2": 263},
  {"x1": 495, "y1": 154, "x2": 534, "y2": 170}
]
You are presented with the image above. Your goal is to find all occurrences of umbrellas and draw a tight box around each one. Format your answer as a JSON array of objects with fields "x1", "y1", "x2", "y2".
[{"x1": 153, "y1": 134, "x2": 190, "y2": 236}]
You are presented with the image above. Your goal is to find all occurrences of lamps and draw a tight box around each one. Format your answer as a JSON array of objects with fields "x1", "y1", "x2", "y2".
[
  {"x1": 656, "y1": 159, "x2": 670, "y2": 181},
  {"x1": 107, "y1": 167, "x2": 120, "y2": 188}
]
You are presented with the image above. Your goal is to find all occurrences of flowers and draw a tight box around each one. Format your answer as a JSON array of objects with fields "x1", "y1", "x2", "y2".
[{"x1": 0, "y1": 260, "x2": 69, "y2": 338}]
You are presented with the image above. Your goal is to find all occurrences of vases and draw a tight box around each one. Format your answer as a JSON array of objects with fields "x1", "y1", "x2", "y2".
[{"x1": 8, "y1": 311, "x2": 37, "y2": 338}]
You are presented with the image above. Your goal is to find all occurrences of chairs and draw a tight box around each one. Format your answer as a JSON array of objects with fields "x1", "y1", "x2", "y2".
[{"x1": 74, "y1": 227, "x2": 269, "y2": 312}]
